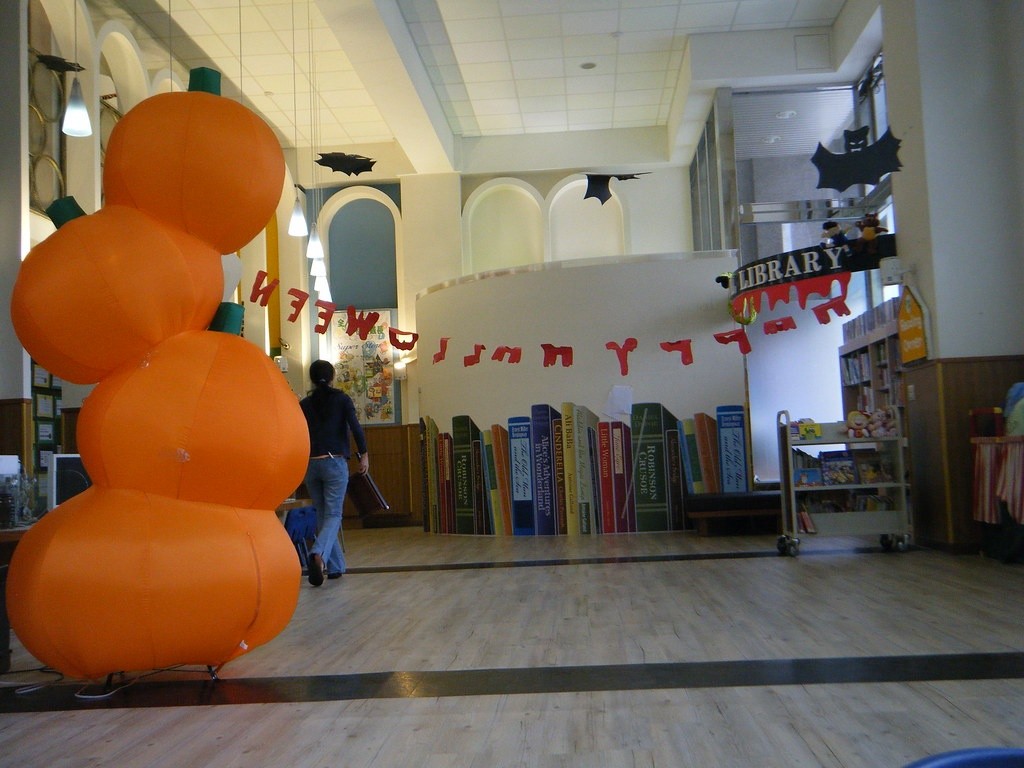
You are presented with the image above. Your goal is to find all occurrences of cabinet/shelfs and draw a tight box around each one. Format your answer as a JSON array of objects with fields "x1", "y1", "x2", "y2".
[
  {"x1": 840, "y1": 318, "x2": 908, "y2": 470},
  {"x1": 775, "y1": 404, "x2": 911, "y2": 556}
]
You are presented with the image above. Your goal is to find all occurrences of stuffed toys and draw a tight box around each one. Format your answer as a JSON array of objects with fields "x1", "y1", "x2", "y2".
[
  {"x1": 846, "y1": 411, "x2": 870, "y2": 438},
  {"x1": 868, "y1": 409, "x2": 898, "y2": 436},
  {"x1": 854, "y1": 213, "x2": 888, "y2": 243}
]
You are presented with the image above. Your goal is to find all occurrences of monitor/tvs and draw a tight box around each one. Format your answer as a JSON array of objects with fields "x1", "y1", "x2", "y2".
[{"x1": 46, "y1": 454, "x2": 93, "y2": 511}]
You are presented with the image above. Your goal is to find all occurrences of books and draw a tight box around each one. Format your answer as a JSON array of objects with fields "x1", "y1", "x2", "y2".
[
  {"x1": 888, "y1": 378, "x2": 905, "y2": 406},
  {"x1": 841, "y1": 356, "x2": 860, "y2": 385},
  {"x1": 817, "y1": 451, "x2": 859, "y2": 486},
  {"x1": 419, "y1": 402, "x2": 749, "y2": 536},
  {"x1": 793, "y1": 448, "x2": 819, "y2": 469},
  {"x1": 856, "y1": 384, "x2": 873, "y2": 412},
  {"x1": 859, "y1": 353, "x2": 871, "y2": 380},
  {"x1": 848, "y1": 448, "x2": 888, "y2": 484},
  {"x1": 841, "y1": 297, "x2": 902, "y2": 345},
  {"x1": 795, "y1": 495, "x2": 897, "y2": 513},
  {"x1": 875, "y1": 343, "x2": 889, "y2": 387}
]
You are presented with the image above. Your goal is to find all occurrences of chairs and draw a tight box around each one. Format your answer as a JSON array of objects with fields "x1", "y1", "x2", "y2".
[{"x1": 284, "y1": 499, "x2": 319, "y2": 568}]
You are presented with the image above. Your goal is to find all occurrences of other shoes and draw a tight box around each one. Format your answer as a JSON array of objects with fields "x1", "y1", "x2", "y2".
[
  {"x1": 308, "y1": 553, "x2": 323, "y2": 586},
  {"x1": 328, "y1": 573, "x2": 342, "y2": 579}
]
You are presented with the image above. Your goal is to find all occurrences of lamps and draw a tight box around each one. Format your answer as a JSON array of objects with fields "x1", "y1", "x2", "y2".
[
  {"x1": 61, "y1": 0, "x2": 92, "y2": 138},
  {"x1": 287, "y1": 0, "x2": 332, "y2": 302},
  {"x1": 880, "y1": 255, "x2": 918, "y2": 285}
]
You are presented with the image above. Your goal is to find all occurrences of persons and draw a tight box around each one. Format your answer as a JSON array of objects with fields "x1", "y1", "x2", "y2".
[
  {"x1": 298, "y1": 360, "x2": 369, "y2": 587},
  {"x1": 818, "y1": 221, "x2": 853, "y2": 249}
]
